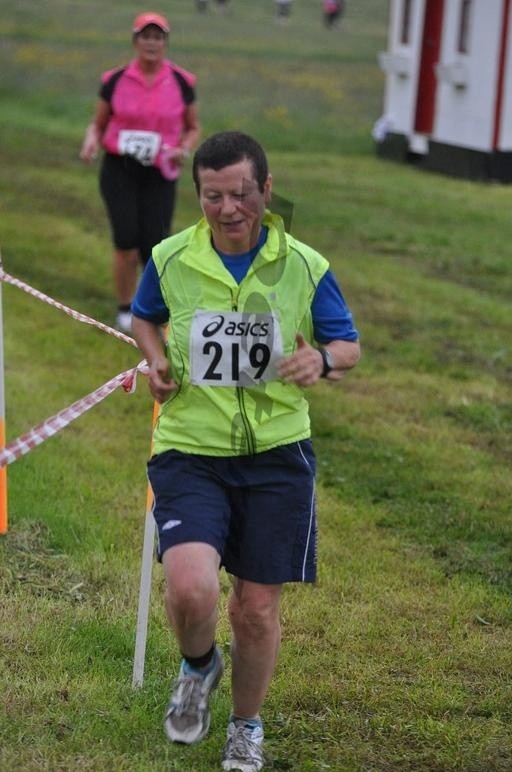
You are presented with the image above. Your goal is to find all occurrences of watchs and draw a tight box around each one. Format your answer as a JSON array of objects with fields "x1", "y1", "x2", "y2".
[{"x1": 314, "y1": 345, "x2": 334, "y2": 378}]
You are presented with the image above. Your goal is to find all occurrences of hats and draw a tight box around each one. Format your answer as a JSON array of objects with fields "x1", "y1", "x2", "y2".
[{"x1": 133, "y1": 13, "x2": 170, "y2": 33}]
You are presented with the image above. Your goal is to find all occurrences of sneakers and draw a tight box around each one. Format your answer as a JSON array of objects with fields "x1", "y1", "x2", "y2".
[
  {"x1": 221, "y1": 720, "x2": 264, "y2": 772},
  {"x1": 164, "y1": 648, "x2": 222, "y2": 745}
]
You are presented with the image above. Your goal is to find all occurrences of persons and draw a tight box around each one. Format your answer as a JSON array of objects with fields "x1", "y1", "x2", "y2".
[
  {"x1": 77, "y1": 10, "x2": 202, "y2": 335},
  {"x1": 128, "y1": 131, "x2": 360, "y2": 772}
]
